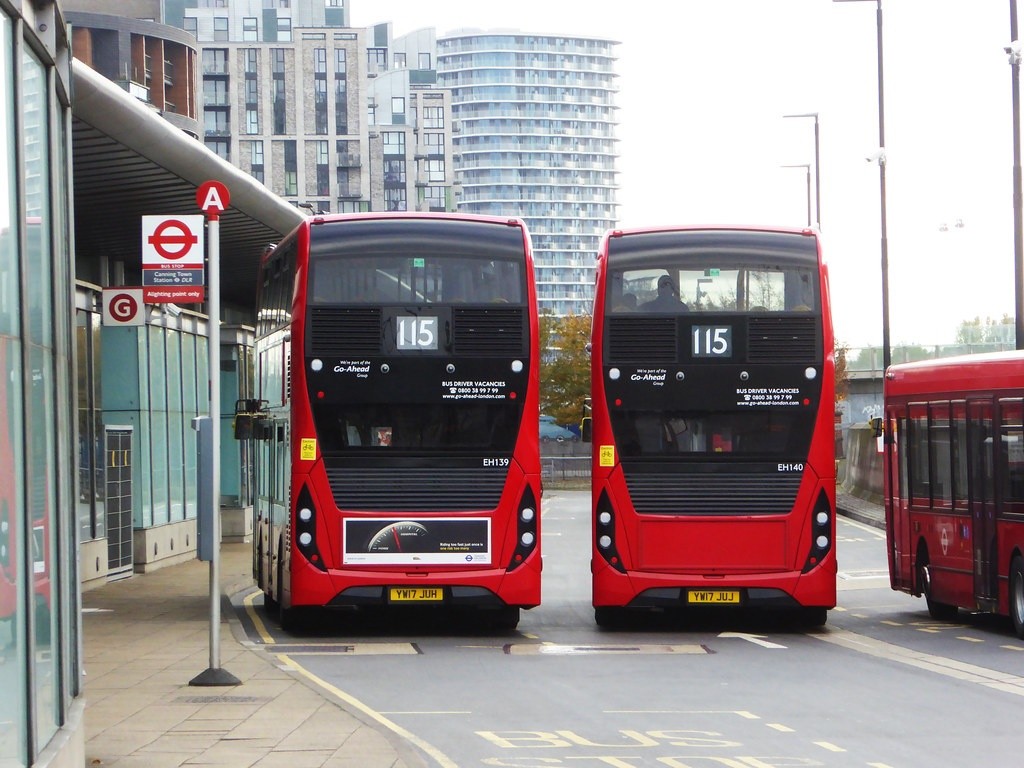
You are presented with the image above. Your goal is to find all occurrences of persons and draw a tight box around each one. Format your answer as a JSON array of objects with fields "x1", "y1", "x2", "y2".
[
  {"x1": 639, "y1": 274, "x2": 690, "y2": 311},
  {"x1": 611, "y1": 293, "x2": 636, "y2": 312}
]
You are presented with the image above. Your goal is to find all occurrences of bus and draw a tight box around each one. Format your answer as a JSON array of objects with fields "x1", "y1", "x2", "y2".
[
  {"x1": 869, "y1": 350, "x2": 1024, "y2": 640},
  {"x1": 576, "y1": 226, "x2": 840, "y2": 634},
  {"x1": 231, "y1": 211, "x2": 543, "y2": 636}
]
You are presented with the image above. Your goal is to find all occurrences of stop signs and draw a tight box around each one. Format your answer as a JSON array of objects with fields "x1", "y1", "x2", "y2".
[{"x1": 140, "y1": 213, "x2": 205, "y2": 305}]
